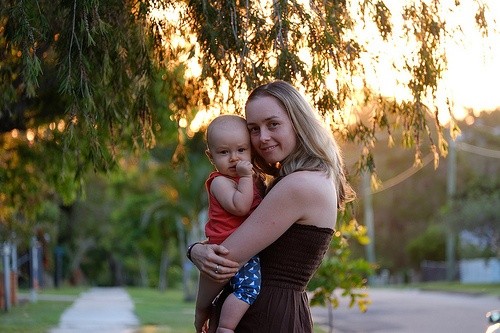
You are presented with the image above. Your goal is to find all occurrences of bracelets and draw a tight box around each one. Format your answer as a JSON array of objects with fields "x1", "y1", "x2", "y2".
[{"x1": 186, "y1": 241, "x2": 204, "y2": 260}]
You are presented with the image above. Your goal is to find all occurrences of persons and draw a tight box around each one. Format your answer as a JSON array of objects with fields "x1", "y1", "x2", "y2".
[
  {"x1": 203, "y1": 115, "x2": 262, "y2": 333},
  {"x1": 194, "y1": 82, "x2": 356, "y2": 333}
]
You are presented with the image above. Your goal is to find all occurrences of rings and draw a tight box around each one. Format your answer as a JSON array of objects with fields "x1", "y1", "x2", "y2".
[{"x1": 215, "y1": 264, "x2": 220, "y2": 274}]
